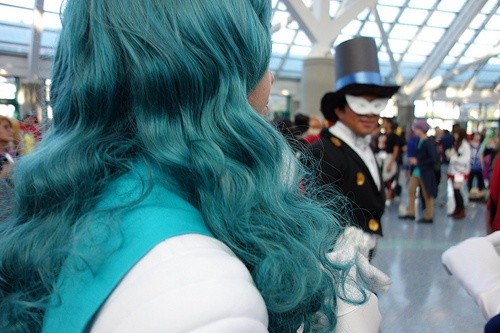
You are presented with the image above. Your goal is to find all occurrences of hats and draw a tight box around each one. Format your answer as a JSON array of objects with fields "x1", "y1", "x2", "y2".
[{"x1": 320, "y1": 36, "x2": 401, "y2": 123}]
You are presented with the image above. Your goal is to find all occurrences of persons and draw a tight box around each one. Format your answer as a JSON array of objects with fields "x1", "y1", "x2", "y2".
[
  {"x1": 262, "y1": 112, "x2": 500, "y2": 235},
  {"x1": 440, "y1": 229, "x2": 500, "y2": 333},
  {"x1": 306, "y1": 36, "x2": 401, "y2": 299},
  {"x1": 0, "y1": 0, "x2": 381, "y2": 332},
  {"x1": 0, "y1": 112, "x2": 43, "y2": 165}
]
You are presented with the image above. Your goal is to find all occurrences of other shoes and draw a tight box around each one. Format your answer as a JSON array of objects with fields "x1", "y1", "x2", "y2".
[
  {"x1": 448, "y1": 208, "x2": 466, "y2": 219},
  {"x1": 417, "y1": 218, "x2": 433, "y2": 224},
  {"x1": 398, "y1": 214, "x2": 415, "y2": 221}
]
